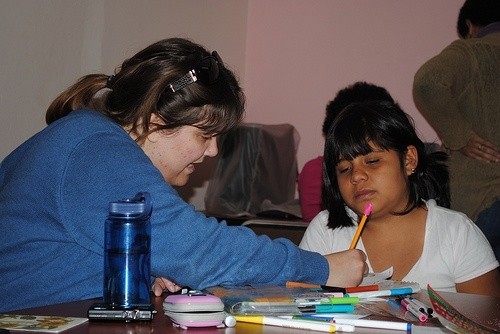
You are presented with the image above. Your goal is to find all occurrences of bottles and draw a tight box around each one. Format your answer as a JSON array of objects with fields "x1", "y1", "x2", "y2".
[{"x1": 101, "y1": 191, "x2": 154, "y2": 311}]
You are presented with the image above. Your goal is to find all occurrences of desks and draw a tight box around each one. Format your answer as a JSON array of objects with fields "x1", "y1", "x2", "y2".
[{"x1": 0, "y1": 289, "x2": 500, "y2": 334}]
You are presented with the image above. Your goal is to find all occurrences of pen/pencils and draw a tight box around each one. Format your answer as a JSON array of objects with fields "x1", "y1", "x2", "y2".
[
  {"x1": 224, "y1": 310, "x2": 236, "y2": 327},
  {"x1": 344, "y1": 285, "x2": 381, "y2": 291},
  {"x1": 278, "y1": 313, "x2": 355, "y2": 332},
  {"x1": 344, "y1": 287, "x2": 413, "y2": 298},
  {"x1": 391, "y1": 292, "x2": 435, "y2": 320},
  {"x1": 348, "y1": 202, "x2": 372, "y2": 251},
  {"x1": 297, "y1": 304, "x2": 357, "y2": 312},
  {"x1": 233, "y1": 316, "x2": 335, "y2": 333},
  {"x1": 333, "y1": 317, "x2": 413, "y2": 329},
  {"x1": 286, "y1": 281, "x2": 346, "y2": 293}
]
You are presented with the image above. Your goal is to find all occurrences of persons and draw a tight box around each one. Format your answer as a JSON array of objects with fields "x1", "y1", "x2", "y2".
[
  {"x1": 412, "y1": 0, "x2": 500, "y2": 261},
  {"x1": 299, "y1": 100, "x2": 500, "y2": 296},
  {"x1": 299, "y1": 81, "x2": 394, "y2": 221},
  {"x1": 0, "y1": 37, "x2": 369, "y2": 311}
]
